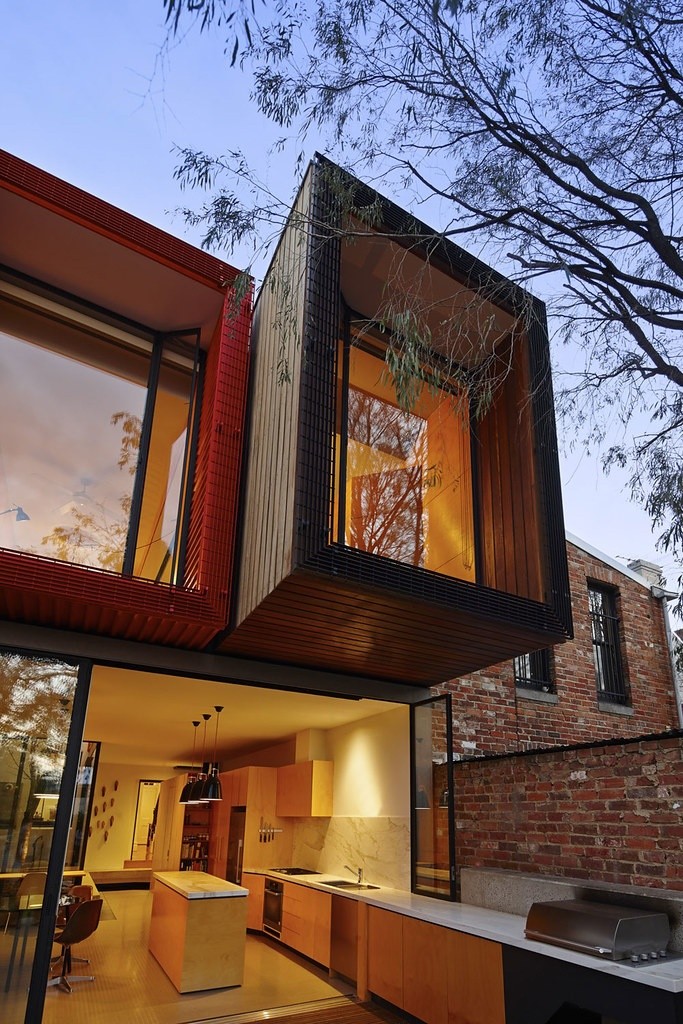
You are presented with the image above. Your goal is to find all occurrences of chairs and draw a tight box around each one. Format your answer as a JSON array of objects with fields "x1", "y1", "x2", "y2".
[{"x1": 14, "y1": 871, "x2": 104, "y2": 993}]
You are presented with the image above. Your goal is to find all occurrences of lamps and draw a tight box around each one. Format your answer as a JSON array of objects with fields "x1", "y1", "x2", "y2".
[
  {"x1": 33, "y1": 698, "x2": 69, "y2": 799},
  {"x1": 179, "y1": 706, "x2": 226, "y2": 805},
  {"x1": 0, "y1": 507, "x2": 31, "y2": 521}
]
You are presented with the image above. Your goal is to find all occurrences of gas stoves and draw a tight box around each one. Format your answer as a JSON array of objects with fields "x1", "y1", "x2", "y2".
[{"x1": 268, "y1": 868, "x2": 323, "y2": 876}]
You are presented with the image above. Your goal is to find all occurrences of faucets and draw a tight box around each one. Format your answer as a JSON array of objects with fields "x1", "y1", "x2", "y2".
[{"x1": 344, "y1": 865, "x2": 364, "y2": 883}]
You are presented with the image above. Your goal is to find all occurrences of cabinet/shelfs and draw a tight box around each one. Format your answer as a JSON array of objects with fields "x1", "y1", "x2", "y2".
[
  {"x1": 242, "y1": 866, "x2": 683, "y2": 1023},
  {"x1": 152, "y1": 765, "x2": 292, "y2": 886}
]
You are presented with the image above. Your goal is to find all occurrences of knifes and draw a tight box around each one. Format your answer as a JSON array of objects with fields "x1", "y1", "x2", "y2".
[{"x1": 259, "y1": 816, "x2": 272, "y2": 842}]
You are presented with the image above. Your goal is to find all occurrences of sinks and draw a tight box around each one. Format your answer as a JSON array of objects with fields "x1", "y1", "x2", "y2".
[
  {"x1": 336, "y1": 885, "x2": 381, "y2": 891},
  {"x1": 316, "y1": 879, "x2": 362, "y2": 887}
]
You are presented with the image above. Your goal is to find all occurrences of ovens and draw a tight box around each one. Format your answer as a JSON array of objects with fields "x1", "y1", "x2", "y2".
[{"x1": 262, "y1": 878, "x2": 284, "y2": 941}]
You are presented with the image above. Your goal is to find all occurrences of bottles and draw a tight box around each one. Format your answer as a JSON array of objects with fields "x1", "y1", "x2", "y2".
[{"x1": 180, "y1": 831, "x2": 209, "y2": 874}]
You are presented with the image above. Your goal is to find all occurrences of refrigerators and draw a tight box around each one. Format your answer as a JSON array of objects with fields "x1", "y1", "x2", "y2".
[{"x1": 226, "y1": 807, "x2": 247, "y2": 886}]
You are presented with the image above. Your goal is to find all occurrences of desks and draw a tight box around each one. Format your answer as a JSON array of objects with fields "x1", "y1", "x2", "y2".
[
  {"x1": 148, "y1": 871, "x2": 250, "y2": 994},
  {"x1": 0, "y1": 894, "x2": 83, "y2": 993}
]
